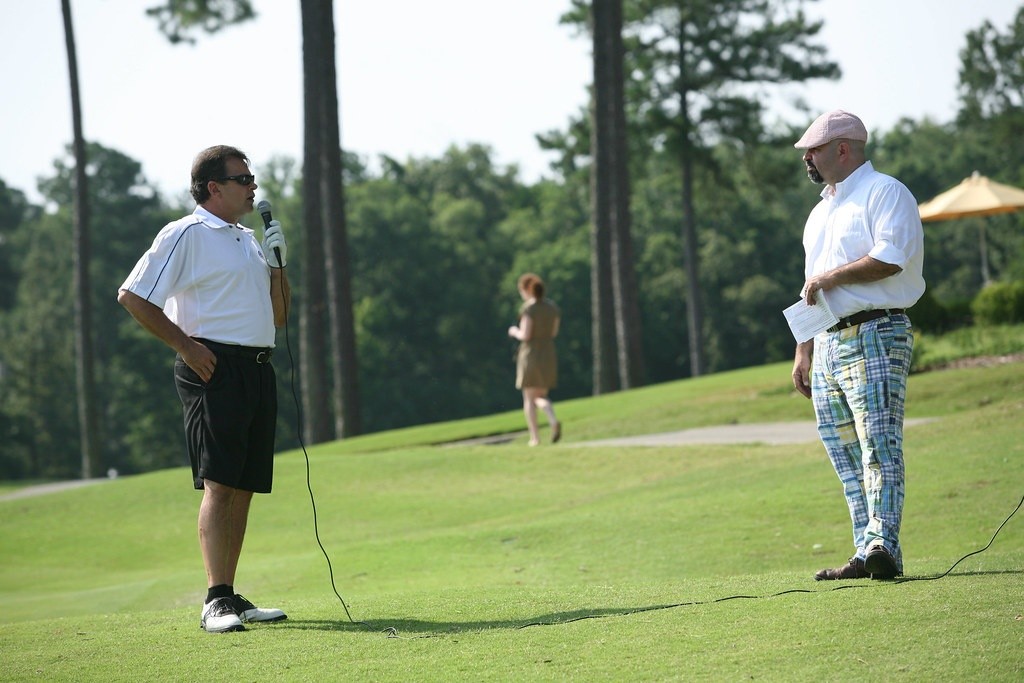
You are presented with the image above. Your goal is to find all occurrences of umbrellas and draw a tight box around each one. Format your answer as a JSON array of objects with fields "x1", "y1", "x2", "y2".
[{"x1": 915, "y1": 172, "x2": 1023, "y2": 285}]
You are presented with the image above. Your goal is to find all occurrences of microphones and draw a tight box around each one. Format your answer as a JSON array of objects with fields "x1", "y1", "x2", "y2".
[{"x1": 257, "y1": 200, "x2": 283, "y2": 268}]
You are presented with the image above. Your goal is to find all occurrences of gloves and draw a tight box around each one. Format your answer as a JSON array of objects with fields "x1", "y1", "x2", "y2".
[{"x1": 261, "y1": 219, "x2": 287, "y2": 269}]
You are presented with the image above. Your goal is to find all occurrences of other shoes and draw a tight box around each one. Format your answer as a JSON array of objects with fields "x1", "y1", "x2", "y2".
[{"x1": 550, "y1": 422, "x2": 562, "y2": 444}]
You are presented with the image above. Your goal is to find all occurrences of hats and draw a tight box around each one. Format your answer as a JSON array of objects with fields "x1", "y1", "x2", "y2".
[{"x1": 793, "y1": 108, "x2": 868, "y2": 148}]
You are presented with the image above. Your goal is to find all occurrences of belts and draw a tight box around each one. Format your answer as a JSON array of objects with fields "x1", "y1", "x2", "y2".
[
  {"x1": 197, "y1": 339, "x2": 275, "y2": 365},
  {"x1": 823, "y1": 307, "x2": 905, "y2": 332}
]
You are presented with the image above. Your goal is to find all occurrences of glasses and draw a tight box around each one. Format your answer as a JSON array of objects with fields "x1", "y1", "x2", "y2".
[{"x1": 222, "y1": 174, "x2": 255, "y2": 185}]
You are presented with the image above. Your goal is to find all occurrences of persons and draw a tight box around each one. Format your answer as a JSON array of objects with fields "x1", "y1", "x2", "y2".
[
  {"x1": 508, "y1": 274, "x2": 561, "y2": 446},
  {"x1": 117, "y1": 144, "x2": 290, "y2": 631}
]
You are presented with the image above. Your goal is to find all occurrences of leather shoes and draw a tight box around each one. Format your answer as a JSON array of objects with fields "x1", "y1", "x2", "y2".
[
  {"x1": 865, "y1": 545, "x2": 899, "y2": 579},
  {"x1": 815, "y1": 557, "x2": 873, "y2": 580},
  {"x1": 199, "y1": 597, "x2": 246, "y2": 633},
  {"x1": 231, "y1": 593, "x2": 288, "y2": 622}
]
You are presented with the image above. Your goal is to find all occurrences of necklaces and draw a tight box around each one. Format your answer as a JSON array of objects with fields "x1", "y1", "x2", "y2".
[{"x1": 792, "y1": 110, "x2": 924, "y2": 581}]
